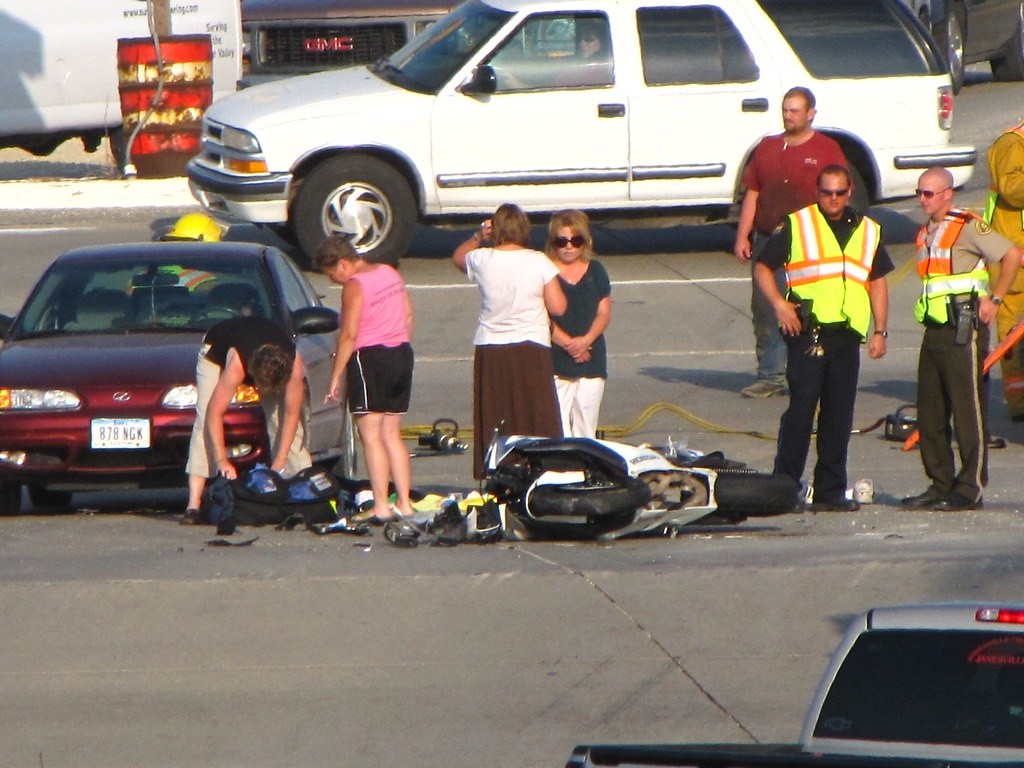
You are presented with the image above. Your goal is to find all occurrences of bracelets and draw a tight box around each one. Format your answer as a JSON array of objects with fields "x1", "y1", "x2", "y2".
[
  {"x1": 276, "y1": 456, "x2": 289, "y2": 460},
  {"x1": 216, "y1": 456, "x2": 229, "y2": 464},
  {"x1": 474, "y1": 230, "x2": 484, "y2": 244}
]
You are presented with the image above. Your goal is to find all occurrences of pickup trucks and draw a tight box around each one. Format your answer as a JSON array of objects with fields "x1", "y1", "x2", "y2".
[
  {"x1": 0, "y1": 0, "x2": 242, "y2": 172},
  {"x1": 183, "y1": 0, "x2": 978, "y2": 267},
  {"x1": 565, "y1": 600, "x2": 1023, "y2": 766}
]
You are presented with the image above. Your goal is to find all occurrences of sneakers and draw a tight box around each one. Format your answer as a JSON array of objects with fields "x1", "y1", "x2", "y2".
[{"x1": 743, "y1": 379, "x2": 787, "y2": 399}]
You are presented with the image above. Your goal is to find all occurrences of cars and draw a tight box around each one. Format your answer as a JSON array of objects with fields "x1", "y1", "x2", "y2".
[
  {"x1": 238, "y1": 1, "x2": 610, "y2": 83},
  {"x1": 0, "y1": 238, "x2": 357, "y2": 515},
  {"x1": 901, "y1": 0, "x2": 1023, "y2": 96}
]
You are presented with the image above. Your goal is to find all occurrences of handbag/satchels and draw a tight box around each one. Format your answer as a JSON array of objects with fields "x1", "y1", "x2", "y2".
[{"x1": 202, "y1": 466, "x2": 344, "y2": 529}]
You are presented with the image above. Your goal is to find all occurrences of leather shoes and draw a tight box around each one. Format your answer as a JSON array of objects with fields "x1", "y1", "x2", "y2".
[
  {"x1": 813, "y1": 493, "x2": 860, "y2": 512},
  {"x1": 903, "y1": 489, "x2": 985, "y2": 512}
]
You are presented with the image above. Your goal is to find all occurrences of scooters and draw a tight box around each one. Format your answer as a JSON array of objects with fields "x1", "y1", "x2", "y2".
[{"x1": 479, "y1": 422, "x2": 796, "y2": 543}]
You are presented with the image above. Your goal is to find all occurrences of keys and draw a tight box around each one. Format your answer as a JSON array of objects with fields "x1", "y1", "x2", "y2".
[
  {"x1": 754, "y1": 165, "x2": 895, "y2": 511},
  {"x1": 804, "y1": 326, "x2": 824, "y2": 357}
]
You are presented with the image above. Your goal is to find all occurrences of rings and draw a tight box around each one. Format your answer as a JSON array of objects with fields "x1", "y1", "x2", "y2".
[
  {"x1": 481, "y1": 223, "x2": 485, "y2": 228},
  {"x1": 227, "y1": 469, "x2": 232, "y2": 473},
  {"x1": 327, "y1": 394, "x2": 332, "y2": 398}
]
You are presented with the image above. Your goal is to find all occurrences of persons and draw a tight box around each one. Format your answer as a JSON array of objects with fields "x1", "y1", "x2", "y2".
[
  {"x1": 181, "y1": 316, "x2": 312, "y2": 526},
  {"x1": 453, "y1": 203, "x2": 567, "y2": 480},
  {"x1": 902, "y1": 169, "x2": 1020, "y2": 512},
  {"x1": 127, "y1": 213, "x2": 225, "y2": 292},
  {"x1": 497, "y1": 23, "x2": 609, "y2": 92},
  {"x1": 983, "y1": 122, "x2": 1024, "y2": 450},
  {"x1": 736, "y1": 86, "x2": 854, "y2": 399},
  {"x1": 317, "y1": 237, "x2": 414, "y2": 525},
  {"x1": 545, "y1": 209, "x2": 611, "y2": 439}
]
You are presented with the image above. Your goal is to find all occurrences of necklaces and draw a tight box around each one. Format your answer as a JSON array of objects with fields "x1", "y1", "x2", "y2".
[
  {"x1": 554, "y1": 260, "x2": 587, "y2": 284},
  {"x1": 783, "y1": 134, "x2": 811, "y2": 150}
]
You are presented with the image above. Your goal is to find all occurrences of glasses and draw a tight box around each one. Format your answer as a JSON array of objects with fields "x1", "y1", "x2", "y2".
[
  {"x1": 820, "y1": 187, "x2": 850, "y2": 198},
  {"x1": 579, "y1": 34, "x2": 598, "y2": 43},
  {"x1": 553, "y1": 236, "x2": 586, "y2": 248},
  {"x1": 915, "y1": 187, "x2": 951, "y2": 198}
]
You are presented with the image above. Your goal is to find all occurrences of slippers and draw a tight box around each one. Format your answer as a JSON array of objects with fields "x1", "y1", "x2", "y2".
[{"x1": 352, "y1": 507, "x2": 412, "y2": 523}]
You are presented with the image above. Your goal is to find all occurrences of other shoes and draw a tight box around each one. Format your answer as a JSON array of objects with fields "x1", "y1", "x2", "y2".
[{"x1": 180, "y1": 510, "x2": 198, "y2": 525}]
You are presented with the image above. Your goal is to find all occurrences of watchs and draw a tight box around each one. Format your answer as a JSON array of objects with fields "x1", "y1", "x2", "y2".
[
  {"x1": 874, "y1": 330, "x2": 888, "y2": 338},
  {"x1": 990, "y1": 294, "x2": 1004, "y2": 305}
]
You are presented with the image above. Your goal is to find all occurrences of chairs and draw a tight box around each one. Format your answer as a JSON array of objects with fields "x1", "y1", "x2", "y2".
[
  {"x1": 206, "y1": 283, "x2": 261, "y2": 318},
  {"x1": 63, "y1": 289, "x2": 131, "y2": 331}
]
